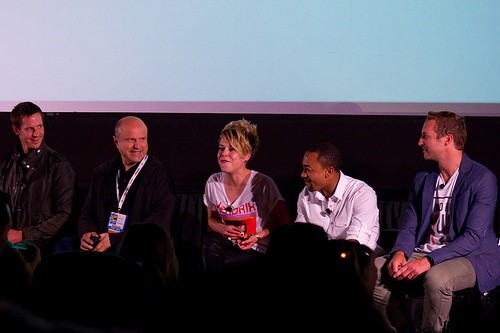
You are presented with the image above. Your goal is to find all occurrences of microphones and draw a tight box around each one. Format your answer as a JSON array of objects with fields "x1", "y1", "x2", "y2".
[
  {"x1": 440, "y1": 184, "x2": 445, "y2": 189},
  {"x1": 226, "y1": 207, "x2": 232, "y2": 212},
  {"x1": 326, "y1": 208, "x2": 330, "y2": 214}
]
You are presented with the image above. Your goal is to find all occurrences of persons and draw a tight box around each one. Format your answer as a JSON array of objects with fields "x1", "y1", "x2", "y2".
[
  {"x1": 0, "y1": 189, "x2": 42, "y2": 308},
  {"x1": 201, "y1": 117, "x2": 292, "y2": 284},
  {"x1": 296, "y1": 142, "x2": 381, "y2": 259},
  {"x1": 0, "y1": 222, "x2": 393, "y2": 333},
  {"x1": 75, "y1": 115, "x2": 180, "y2": 260},
  {"x1": 0, "y1": 101, "x2": 74, "y2": 255},
  {"x1": 363, "y1": 110, "x2": 500, "y2": 333}
]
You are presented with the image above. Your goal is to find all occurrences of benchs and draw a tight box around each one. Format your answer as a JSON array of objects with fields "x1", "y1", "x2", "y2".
[{"x1": 70, "y1": 188, "x2": 500, "y2": 309}]
